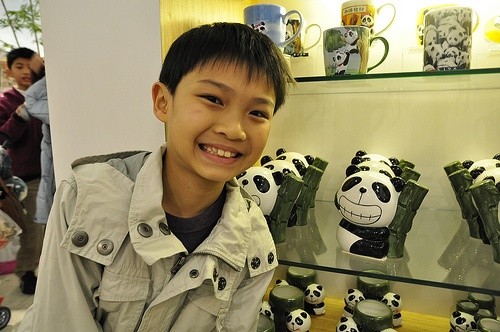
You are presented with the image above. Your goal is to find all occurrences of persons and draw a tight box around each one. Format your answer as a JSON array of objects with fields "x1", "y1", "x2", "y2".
[
  {"x1": 0, "y1": 48, "x2": 40, "y2": 295},
  {"x1": 30, "y1": 52, "x2": 46, "y2": 75},
  {"x1": 20, "y1": 23, "x2": 298, "y2": 328}
]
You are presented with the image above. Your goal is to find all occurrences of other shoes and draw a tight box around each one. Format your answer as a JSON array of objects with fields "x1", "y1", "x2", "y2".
[{"x1": 19, "y1": 273, "x2": 37, "y2": 295}]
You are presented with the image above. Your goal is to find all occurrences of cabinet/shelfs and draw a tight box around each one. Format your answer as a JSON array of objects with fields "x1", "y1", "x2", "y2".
[{"x1": 159, "y1": 0, "x2": 500, "y2": 332}]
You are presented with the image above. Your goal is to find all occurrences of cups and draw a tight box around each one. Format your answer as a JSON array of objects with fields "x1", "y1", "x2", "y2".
[
  {"x1": 417, "y1": 4, "x2": 480, "y2": 47},
  {"x1": 340, "y1": 0, "x2": 397, "y2": 36},
  {"x1": 323, "y1": 26, "x2": 390, "y2": 76},
  {"x1": 283, "y1": 19, "x2": 322, "y2": 54},
  {"x1": 423, "y1": 7, "x2": 472, "y2": 71},
  {"x1": 243, "y1": 4, "x2": 303, "y2": 54}
]
